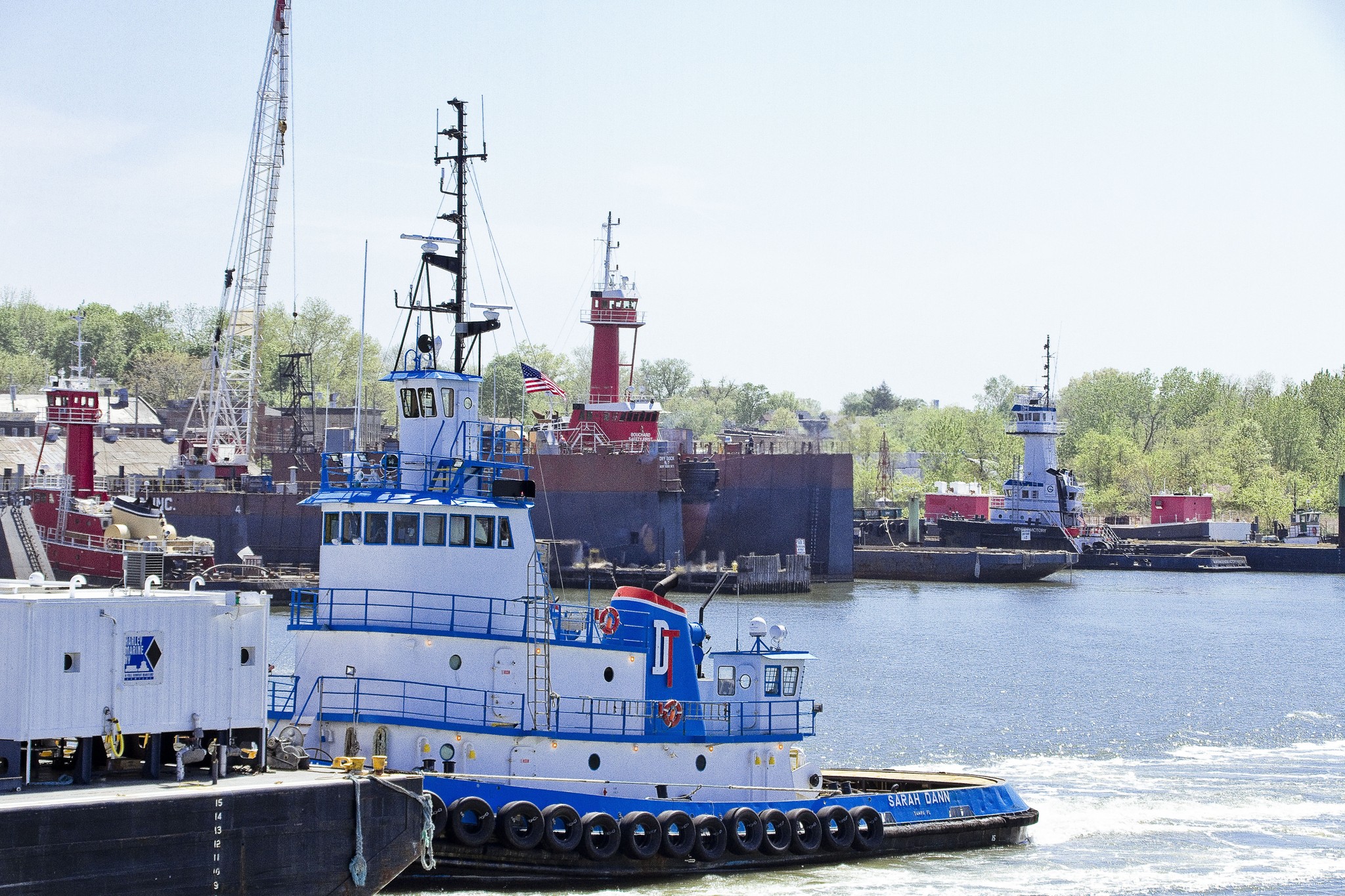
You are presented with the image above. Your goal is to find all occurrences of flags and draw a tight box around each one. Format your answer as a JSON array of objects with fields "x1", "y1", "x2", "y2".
[{"x1": 519, "y1": 360, "x2": 568, "y2": 400}]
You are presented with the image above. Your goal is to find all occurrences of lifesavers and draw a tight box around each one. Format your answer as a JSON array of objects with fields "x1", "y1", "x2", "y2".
[
  {"x1": 663, "y1": 700, "x2": 682, "y2": 728},
  {"x1": 599, "y1": 607, "x2": 620, "y2": 635}
]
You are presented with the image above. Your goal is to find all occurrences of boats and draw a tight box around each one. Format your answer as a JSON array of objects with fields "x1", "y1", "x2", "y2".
[
  {"x1": 270, "y1": 93, "x2": 1044, "y2": 886},
  {"x1": 0, "y1": 0, "x2": 1345, "y2": 609}
]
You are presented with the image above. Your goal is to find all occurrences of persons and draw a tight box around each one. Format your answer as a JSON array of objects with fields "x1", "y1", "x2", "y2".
[{"x1": 747, "y1": 434, "x2": 755, "y2": 454}]
